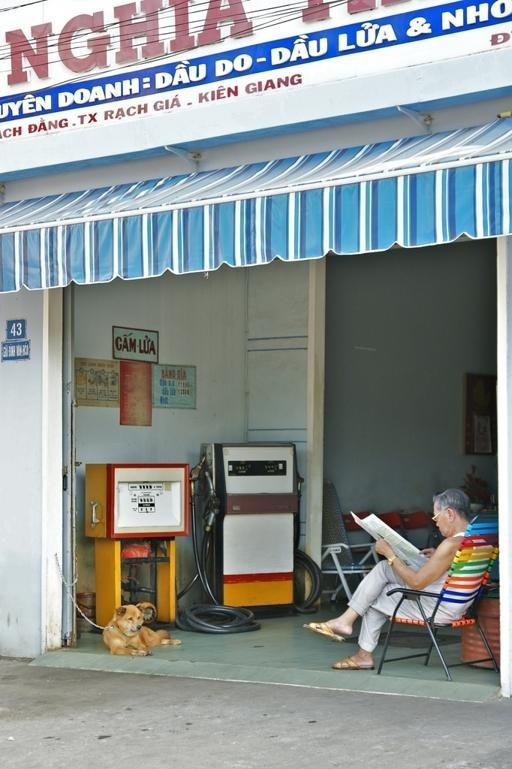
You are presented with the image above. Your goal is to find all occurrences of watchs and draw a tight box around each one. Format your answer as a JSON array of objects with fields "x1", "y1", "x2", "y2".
[{"x1": 388, "y1": 555, "x2": 398, "y2": 566}]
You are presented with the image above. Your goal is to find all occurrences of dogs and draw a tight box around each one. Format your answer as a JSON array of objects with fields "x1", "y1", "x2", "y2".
[{"x1": 102, "y1": 604, "x2": 182, "y2": 657}]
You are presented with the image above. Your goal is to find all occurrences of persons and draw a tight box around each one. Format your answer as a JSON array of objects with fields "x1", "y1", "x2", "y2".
[{"x1": 302, "y1": 488, "x2": 493, "y2": 671}]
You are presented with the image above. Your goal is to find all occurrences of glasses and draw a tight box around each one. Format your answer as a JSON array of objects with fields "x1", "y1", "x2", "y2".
[{"x1": 432, "y1": 505, "x2": 450, "y2": 522}]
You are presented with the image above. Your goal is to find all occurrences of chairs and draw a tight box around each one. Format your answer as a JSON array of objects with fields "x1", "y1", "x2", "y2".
[
  {"x1": 376, "y1": 502, "x2": 499, "y2": 679},
  {"x1": 343, "y1": 510, "x2": 445, "y2": 581},
  {"x1": 320, "y1": 477, "x2": 382, "y2": 603}
]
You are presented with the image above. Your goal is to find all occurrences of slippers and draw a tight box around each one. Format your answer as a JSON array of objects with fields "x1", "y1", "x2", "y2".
[
  {"x1": 332, "y1": 655, "x2": 376, "y2": 670},
  {"x1": 302, "y1": 621, "x2": 345, "y2": 642}
]
